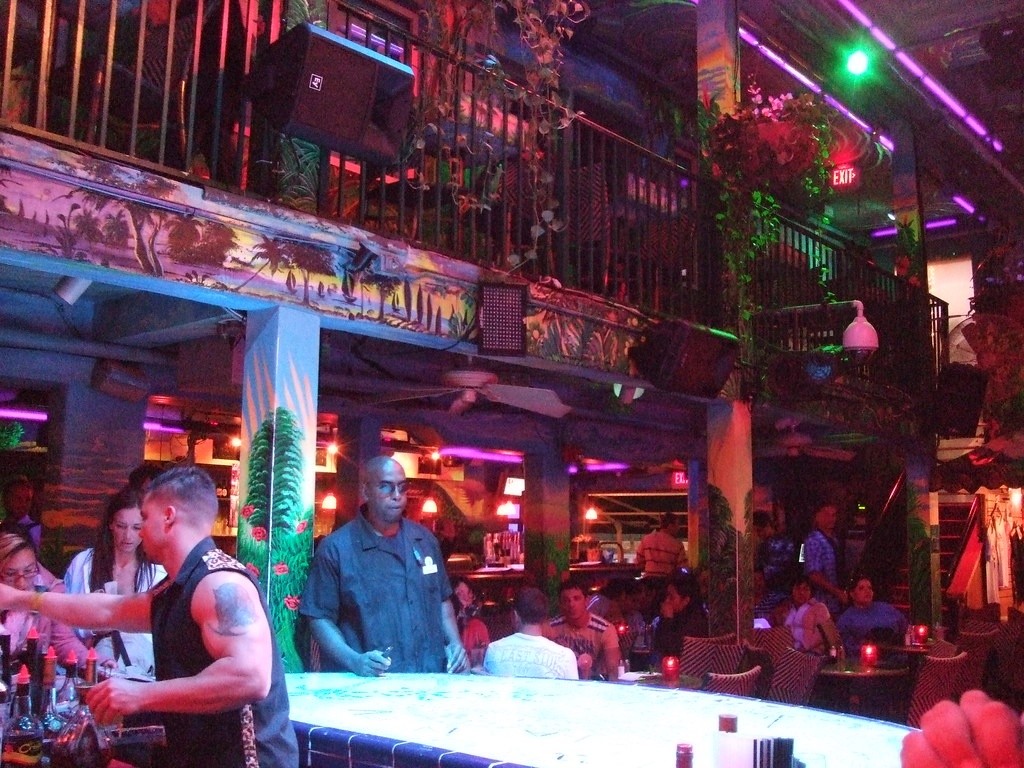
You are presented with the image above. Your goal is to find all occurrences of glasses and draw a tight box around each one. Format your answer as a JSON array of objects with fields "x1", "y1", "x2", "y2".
[
  {"x1": 363, "y1": 480, "x2": 408, "y2": 495},
  {"x1": 0, "y1": 561, "x2": 39, "y2": 583}
]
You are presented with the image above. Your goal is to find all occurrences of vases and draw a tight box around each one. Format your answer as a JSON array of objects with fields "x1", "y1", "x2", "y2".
[{"x1": 700, "y1": 120, "x2": 820, "y2": 183}]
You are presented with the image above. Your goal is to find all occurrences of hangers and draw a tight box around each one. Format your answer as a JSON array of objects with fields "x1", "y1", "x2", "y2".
[{"x1": 985, "y1": 494, "x2": 1003, "y2": 530}]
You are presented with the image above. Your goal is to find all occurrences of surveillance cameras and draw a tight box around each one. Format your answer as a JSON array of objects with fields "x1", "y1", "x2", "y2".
[{"x1": 843, "y1": 316, "x2": 879, "y2": 363}]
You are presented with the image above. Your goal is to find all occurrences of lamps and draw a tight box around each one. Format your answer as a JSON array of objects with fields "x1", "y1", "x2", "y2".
[
  {"x1": 53, "y1": 276, "x2": 92, "y2": 305},
  {"x1": 586, "y1": 494, "x2": 598, "y2": 519},
  {"x1": 422, "y1": 496, "x2": 437, "y2": 513},
  {"x1": 320, "y1": 492, "x2": 337, "y2": 509}
]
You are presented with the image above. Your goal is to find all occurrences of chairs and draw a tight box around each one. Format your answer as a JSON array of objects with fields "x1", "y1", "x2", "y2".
[
  {"x1": 451, "y1": 157, "x2": 839, "y2": 353},
  {"x1": 49, "y1": 0, "x2": 218, "y2": 171},
  {"x1": 478, "y1": 603, "x2": 513, "y2": 642},
  {"x1": 618, "y1": 601, "x2": 1024, "y2": 730}
]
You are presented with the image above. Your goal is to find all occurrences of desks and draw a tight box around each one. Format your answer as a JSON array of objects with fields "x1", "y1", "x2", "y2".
[
  {"x1": 637, "y1": 674, "x2": 704, "y2": 689},
  {"x1": 819, "y1": 658, "x2": 909, "y2": 715},
  {"x1": 284, "y1": 671, "x2": 923, "y2": 768},
  {"x1": 880, "y1": 643, "x2": 932, "y2": 684}
]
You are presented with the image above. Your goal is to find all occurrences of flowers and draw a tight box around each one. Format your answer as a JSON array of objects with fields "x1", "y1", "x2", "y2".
[{"x1": 670, "y1": 72, "x2": 847, "y2": 403}]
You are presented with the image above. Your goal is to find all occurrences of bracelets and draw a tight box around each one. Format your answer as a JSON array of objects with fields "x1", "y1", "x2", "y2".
[{"x1": 28, "y1": 591, "x2": 47, "y2": 614}]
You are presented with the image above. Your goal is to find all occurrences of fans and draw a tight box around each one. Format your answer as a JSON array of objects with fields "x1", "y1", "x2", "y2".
[
  {"x1": 752, "y1": 415, "x2": 856, "y2": 461},
  {"x1": 940, "y1": 312, "x2": 1024, "y2": 402},
  {"x1": 362, "y1": 356, "x2": 574, "y2": 418}
]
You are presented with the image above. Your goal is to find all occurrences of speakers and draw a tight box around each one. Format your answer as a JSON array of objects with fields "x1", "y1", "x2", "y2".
[
  {"x1": 934, "y1": 362, "x2": 987, "y2": 439},
  {"x1": 254, "y1": 22, "x2": 415, "y2": 168},
  {"x1": 91, "y1": 358, "x2": 151, "y2": 401},
  {"x1": 637, "y1": 315, "x2": 741, "y2": 401}
]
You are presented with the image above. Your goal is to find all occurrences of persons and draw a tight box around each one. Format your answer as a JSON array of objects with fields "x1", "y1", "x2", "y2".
[
  {"x1": 473, "y1": 525, "x2": 487, "y2": 565},
  {"x1": 837, "y1": 572, "x2": 908, "y2": 719},
  {"x1": 432, "y1": 519, "x2": 452, "y2": 569},
  {"x1": 784, "y1": 574, "x2": 844, "y2": 659},
  {"x1": 0, "y1": 470, "x2": 301, "y2": 768},
  {"x1": 630, "y1": 578, "x2": 709, "y2": 672},
  {"x1": 64, "y1": 462, "x2": 167, "y2": 678},
  {"x1": 299, "y1": 455, "x2": 470, "y2": 678},
  {"x1": 453, "y1": 570, "x2": 623, "y2": 680},
  {"x1": 752, "y1": 506, "x2": 795, "y2": 591},
  {"x1": 632, "y1": 513, "x2": 688, "y2": 613},
  {"x1": 0, "y1": 474, "x2": 118, "y2": 671},
  {"x1": 805, "y1": 499, "x2": 848, "y2": 624},
  {"x1": 1009, "y1": 519, "x2": 1024, "y2": 606}
]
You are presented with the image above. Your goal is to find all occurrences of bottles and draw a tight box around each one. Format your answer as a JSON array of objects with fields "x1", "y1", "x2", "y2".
[
  {"x1": 8, "y1": 627, "x2": 100, "y2": 733},
  {"x1": 711, "y1": 712, "x2": 745, "y2": 768},
  {"x1": 676, "y1": 744, "x2": 694, "y2": 768}
]
